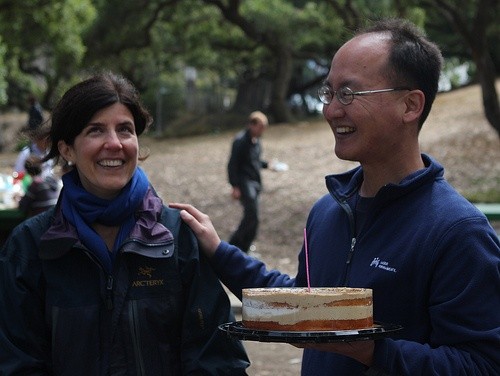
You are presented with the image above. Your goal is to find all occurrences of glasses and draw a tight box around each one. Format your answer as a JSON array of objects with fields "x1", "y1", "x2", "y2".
[{"x1": 318, "y1": 86, "x2": 411, "y2": 105}]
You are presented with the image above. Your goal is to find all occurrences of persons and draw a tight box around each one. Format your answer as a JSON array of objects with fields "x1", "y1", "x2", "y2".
[
  {"x1": 0, "y1": 69, "x2": 254, "y2": 375},
  {"x1": 224, "y1": 110, "x2": 280, "y2": 255},
  {"x1": 169, "y1": 13, "x2": 500, "y2": 376},
  {"x1": 19, "y1": 154, "x2": 63, "y2": 219},
  {"x1": 14, "y1": 126, "x2": 57, "y2": 176},
  {"x1": 22, "y1": 95, "x2": 45, "y2": 138}
]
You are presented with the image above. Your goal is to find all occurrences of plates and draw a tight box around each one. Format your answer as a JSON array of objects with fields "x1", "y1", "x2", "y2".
[{"x1": 218, "y1": 322, "x2": 399, "y2": 343}]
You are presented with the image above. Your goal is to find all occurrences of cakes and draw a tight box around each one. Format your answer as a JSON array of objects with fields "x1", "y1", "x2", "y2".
[{"x1": 241, "y1": 286, "x2": 373, "y2": 332}]
